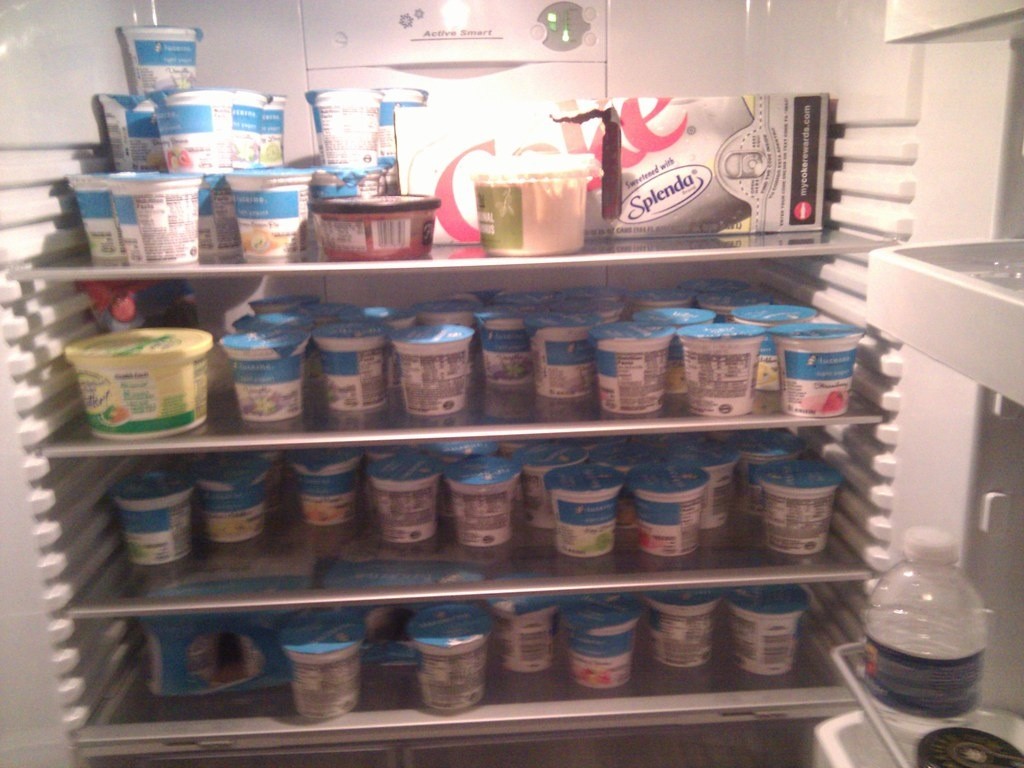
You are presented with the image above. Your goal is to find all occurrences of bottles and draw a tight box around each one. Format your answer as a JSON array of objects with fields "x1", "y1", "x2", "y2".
[
  {"x1": 849, "y1": 526, "x2": 987, "y2": 768},
  {"x1": 912, "y1": 726, "x2": 1024, "y2": 768}
]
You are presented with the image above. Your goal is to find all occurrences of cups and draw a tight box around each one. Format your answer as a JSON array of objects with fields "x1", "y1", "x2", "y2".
[
  {"x1": 289, "y1": 440, "x2": 733, "y2": 559},
  {"x1": 723, "y1": 584, "x2": 809, "y2": 677},
  {"x1": 734, "y1": 430, "x2": 839, "y2": 555},
  {"x1": 65, "y1": 23, "x2": 315, "y2": 264},
  {"x1": 110, "y1": 450, "x2": 269, "y2": 566},
  {"x1": 305, "y1": 86, "x2": 404, "y2": 189},
  {"x1": 219, "y1": 276, "x2": 867, "y2": 418},
  {"x1": 264, "y1": 595, "x2": 644, "y2": 724},
  {"x1": 640, "y1": 590, "x2": 723, "y2": 666}
]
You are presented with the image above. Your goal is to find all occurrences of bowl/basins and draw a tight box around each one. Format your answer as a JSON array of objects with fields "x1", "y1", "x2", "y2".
[
  {"x1": 62, "y1": 326, "x2": 214, "y2": 442},
  {"x1": 311, "y1": 193, "x2": 441, "y2": 260},
  {"x1": 468, "y1": 148, "x2": 600, "y2": 257}
]
